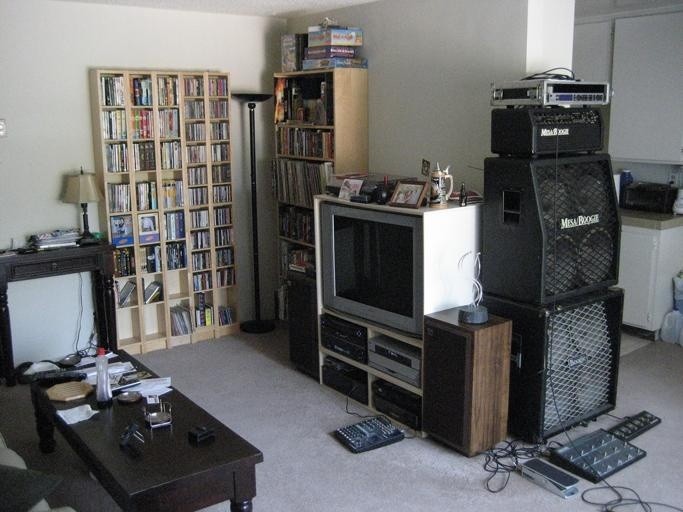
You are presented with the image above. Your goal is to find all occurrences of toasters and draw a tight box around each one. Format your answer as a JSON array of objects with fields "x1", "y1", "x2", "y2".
[{"x1": 623, "y1": 180, "x2": 677, "y2": 213}]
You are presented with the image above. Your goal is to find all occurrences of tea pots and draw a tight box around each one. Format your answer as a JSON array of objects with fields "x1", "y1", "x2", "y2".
[{"x1": 429, "y1": 162, "x2": 453, "y2": 208}]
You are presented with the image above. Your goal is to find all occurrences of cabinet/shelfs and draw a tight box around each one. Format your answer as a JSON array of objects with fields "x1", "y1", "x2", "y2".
[
  {"x1": 86, "y1": 66, "x2": 241, "y2": 357},
  {"x1": 315, "y1": 307, "x2": 424, "y2": 431},
  {"x1": 286, "y1": 271, "x2": 319, "y2": 381},
  {"x1": 571, "y1": 8, "x2": 682, "y2": 166},
  {"x1": 271, "y1": 66, "x2": 370, "y2": 337},
  {"x1": 611, "y1": 225, "x2": 681, "y2": 341}
]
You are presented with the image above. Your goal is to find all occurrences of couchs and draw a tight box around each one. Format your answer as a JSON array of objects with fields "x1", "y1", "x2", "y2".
[{"x1": 0, "y1": 433, "x2": 76, "y2": 512}]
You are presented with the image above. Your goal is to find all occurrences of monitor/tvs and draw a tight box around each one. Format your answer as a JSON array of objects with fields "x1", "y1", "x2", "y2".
[{"x1": 320, "y1": 202, "x2": 424, "y2": 336}]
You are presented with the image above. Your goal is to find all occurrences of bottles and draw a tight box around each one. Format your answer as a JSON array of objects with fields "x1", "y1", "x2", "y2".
[
  {"x1": 619, "y1": 170, "x2": 632, "y2": 206},
  {"x1": 383, "y1": 175, "x2": 390, "y2": 196},
  {"x1": 94, "y1": 348, "x2": 112, "y2": 403},
  {"x1": 459, "y1": 182, "x2": 466, "y2": 207},
  {"x1": 672, "y1": 188, "x2": 682, "y2": 216}
]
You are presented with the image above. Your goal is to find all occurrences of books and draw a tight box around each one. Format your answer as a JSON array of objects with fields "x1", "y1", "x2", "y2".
[
  {"x1": 100, "y1": 76, "x2": 236, "y2": 335},
  {"x1": 271, "y1": 78, "x2": 336, "y2": 280}
]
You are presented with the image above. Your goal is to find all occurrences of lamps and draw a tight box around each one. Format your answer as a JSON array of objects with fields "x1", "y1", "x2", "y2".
[
  {"x1": 59, "y1": 166, "x2": 105, "y2": 248},
  {"x1": 230, "y1": 91, "x2": 279, "y2": 333}
]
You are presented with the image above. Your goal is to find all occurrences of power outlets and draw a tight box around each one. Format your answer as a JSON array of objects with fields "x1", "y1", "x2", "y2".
[{"x1": 668, "y1": 171, "x2": 680, "y2": 188}]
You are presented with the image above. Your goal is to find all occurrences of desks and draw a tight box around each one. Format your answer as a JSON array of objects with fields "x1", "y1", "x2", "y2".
[{"x1": 0, "y1": 240, "x2": 116, "y2": 385}]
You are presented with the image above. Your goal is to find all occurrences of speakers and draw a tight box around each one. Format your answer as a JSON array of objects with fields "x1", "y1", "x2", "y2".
[
  {"x1": 483, "y1": 288, "x2": 624, "y2": 442},
  {"x1": 481, "y1": 159, "x2": 623, "y2": 307},
  {"x1": 491, "y1": 109, "x2": 603, "y2": 158}
]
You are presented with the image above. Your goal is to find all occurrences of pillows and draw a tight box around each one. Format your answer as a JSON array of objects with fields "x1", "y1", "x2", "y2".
[{"x1": 0, "y1": 463, "x2": 64, "y2": 512}]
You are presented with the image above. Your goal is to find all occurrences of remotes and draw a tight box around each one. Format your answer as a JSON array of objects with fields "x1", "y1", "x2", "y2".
[{"x1": 41, "y1": 371, "x2": 87, "y2": 381}]
